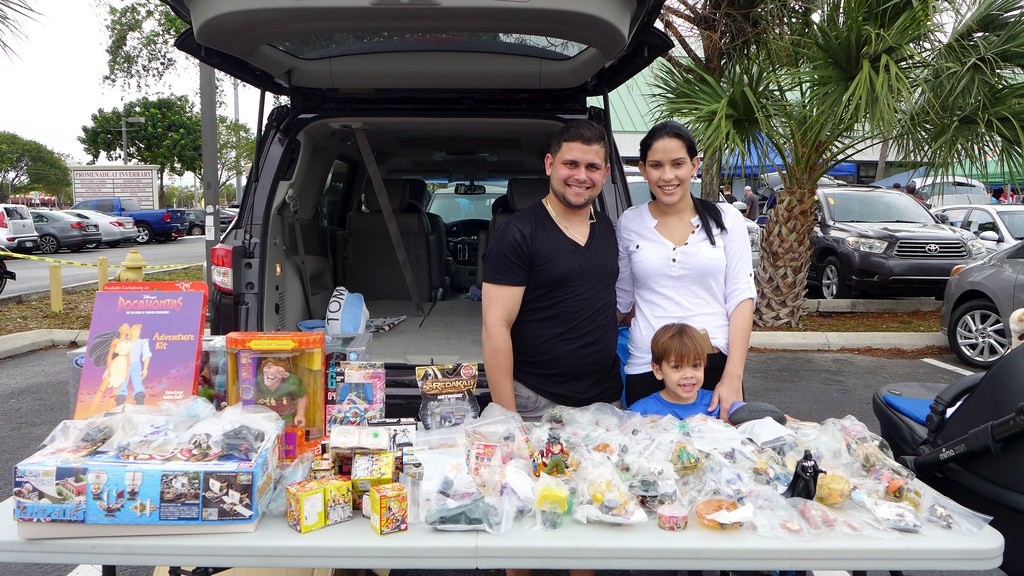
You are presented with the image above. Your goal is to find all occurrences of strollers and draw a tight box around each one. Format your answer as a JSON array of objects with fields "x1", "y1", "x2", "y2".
[{"x1": 871, "y1": 342, "x2": 1024, "y2": 576}]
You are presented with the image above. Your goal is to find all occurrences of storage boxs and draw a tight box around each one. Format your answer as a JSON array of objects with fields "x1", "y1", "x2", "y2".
[{"x1": 12, "y1": 281, "x2": 419, "y2": 536}]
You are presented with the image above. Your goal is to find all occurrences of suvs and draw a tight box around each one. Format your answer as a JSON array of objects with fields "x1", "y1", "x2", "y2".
[{"x1": 619, "y1": 161, "x2": 763, "y2": 273}]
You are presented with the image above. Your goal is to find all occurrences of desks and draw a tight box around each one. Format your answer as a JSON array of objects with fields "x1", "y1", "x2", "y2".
[{"x1": 0, "y1": 472, "x2": 1005, "y2": 576}]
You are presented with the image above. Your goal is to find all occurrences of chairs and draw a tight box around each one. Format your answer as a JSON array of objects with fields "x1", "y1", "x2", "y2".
[
  {"x1": 340, "y1": 174, "x2": 446, "y2": 299},
  {"x1": 477, "y1": 177, "x2": 550, "y2": 287}
]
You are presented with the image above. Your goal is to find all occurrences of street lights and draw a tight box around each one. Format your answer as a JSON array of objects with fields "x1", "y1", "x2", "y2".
[{"x1": 121, "y1": 116, "x2": 147, "y2": 162}]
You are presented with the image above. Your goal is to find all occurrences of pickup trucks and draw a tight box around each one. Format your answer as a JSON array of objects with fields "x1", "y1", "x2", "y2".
[{"x1": 64, "y1": 197, "x2": 191, "y2": 244}]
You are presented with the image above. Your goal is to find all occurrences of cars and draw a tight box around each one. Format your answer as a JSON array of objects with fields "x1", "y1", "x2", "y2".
[
  {"x1": 156, "y1": 0, "x2": 679, "y2": 442},
  {"x1": 184, "y1": 206, "x2": 240, "y2": 237},
  {"x1": 0, "y1": 203, "x2": 40, "y2": 257},
  {"x1": 910, "y1": 175, "x2": 1024, "y2": 256},
  {"x1": 28, "y1": 209, "x2": 104, "y2": 254},
  {"x1": 752, "y1": 169, "x2": 990, "y2": 302},
  {"x1": 939, "y1": 238, "x2": 1024, "y2": 370},
  {"x1": 57, "y1": 209, "x2": 140, "y2": 249},
  {"x1": 424, "y1": 181, "x2": 509, "y2": 226}
]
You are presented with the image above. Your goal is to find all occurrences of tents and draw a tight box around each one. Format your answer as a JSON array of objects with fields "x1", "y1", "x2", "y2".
[
  {"x1": 927, "y1": 146, "x2": 1024, "y2": 185},
  {"x1": 871, "y1": 166, "x2": 929, "y2": 188},
  {"x1": 697, "y1": 131, "x2": 856, "y2": 183}
]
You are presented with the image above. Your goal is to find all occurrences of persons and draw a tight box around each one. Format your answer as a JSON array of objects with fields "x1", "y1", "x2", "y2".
[
  {"x1": 483, "y1": 123, "x2": 624, "y2": 576},
  {"x1": 744, "y1": 186, "x2": 758, "y2": 221},
  {"x1": 199, "y1": 376, "x2": 210, "y2": 398},
  {"x1": 257, "y1": 360, "x2": 306, "y2": 426},
  {"x1": 724, "y1": 185, "x2": 737, "y2": 204},
  {"x1": 630, "y1": 325, "x2": 719, "y2": 576},
  {"x1": 988, "y1": 185, "x2": 1024, "y2": 204},
  {"x1": 542, "y1": 433, "x2": 568, "y2": 475},
  {"x1": 892, "y1": 182, "x2": 926, "y2": 204},
  {"x1": 781, "y1": 449, "x2": 827, "y2": 500},
  {"x1": 616, "y1": 120, "x2": 756, "y2": 576}
]
